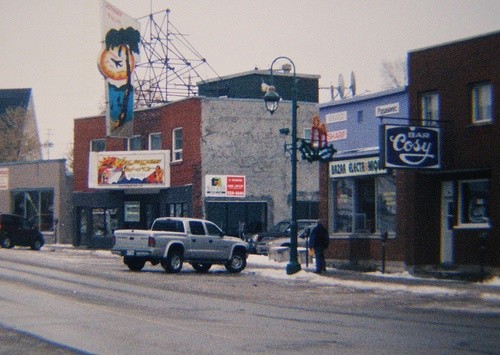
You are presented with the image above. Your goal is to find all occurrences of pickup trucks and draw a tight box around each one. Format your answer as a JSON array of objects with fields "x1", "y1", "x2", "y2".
[{"x1": 113, "y1": 217, "x2": 250, "y2": 274}]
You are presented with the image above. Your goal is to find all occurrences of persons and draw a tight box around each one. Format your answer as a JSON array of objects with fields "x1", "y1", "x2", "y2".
[{"x1": 308, "y1": 219, "x2": 331, "y2": 275}]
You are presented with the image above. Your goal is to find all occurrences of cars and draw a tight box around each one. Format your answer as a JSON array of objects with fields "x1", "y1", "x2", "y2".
[
  {"x1": 257, "y1": 218, "x2": 312, "y2": 254},
  {"x1": 0, "y1": 212, "x2": 45, "y2": 249},
  {"x1": 266, "y1": 220, "x2": 318, "y2": 255}
]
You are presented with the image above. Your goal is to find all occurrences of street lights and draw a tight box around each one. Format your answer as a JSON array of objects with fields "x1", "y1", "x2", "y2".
[{"x1": 264, "y1": 56, "x2": 301, "y2": 274}]
